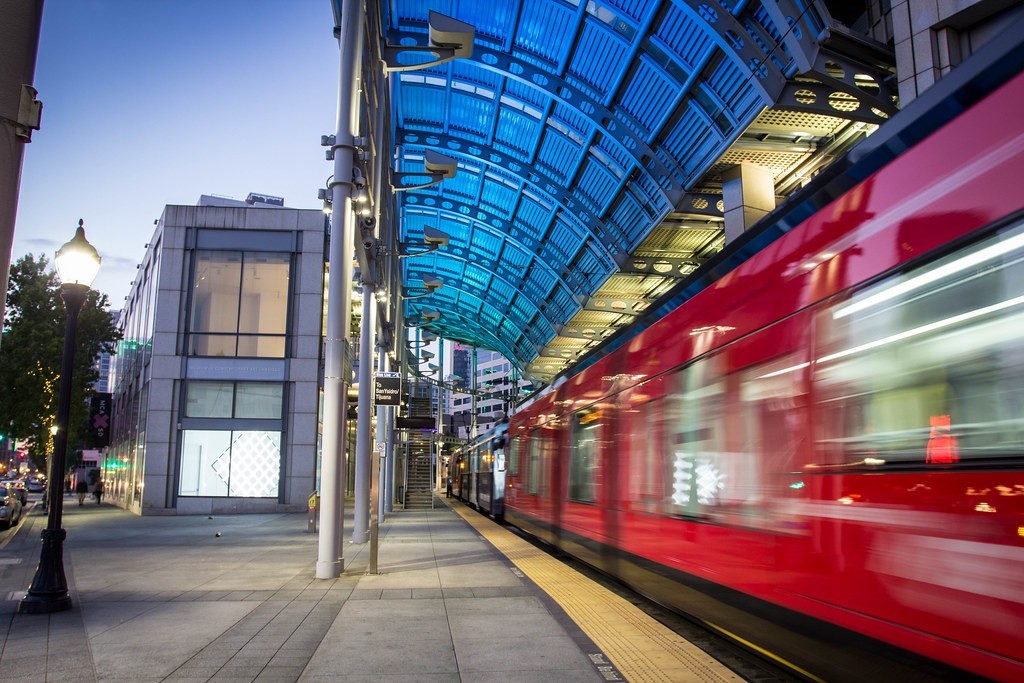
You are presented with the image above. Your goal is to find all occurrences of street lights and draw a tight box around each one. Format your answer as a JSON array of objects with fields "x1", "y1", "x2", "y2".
[{"x1": 19, "y1": 216, "x2": 102, "y2": 617}]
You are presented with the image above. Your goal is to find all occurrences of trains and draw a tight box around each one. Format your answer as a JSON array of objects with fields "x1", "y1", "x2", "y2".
[{"x1": 448, "y1": 15, "x2": 1024, "y2": 682}]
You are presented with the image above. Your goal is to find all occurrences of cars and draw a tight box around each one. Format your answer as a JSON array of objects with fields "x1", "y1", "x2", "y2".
[
  {"x1": 29, "y1": 478, "x2": 44, "y2": 492},
  {"x1": 0, "y1": 480, "x2": 29, "y2": 506},
  {"x1": 0, "y1": 487, "x2": 22, "y2": 530}
]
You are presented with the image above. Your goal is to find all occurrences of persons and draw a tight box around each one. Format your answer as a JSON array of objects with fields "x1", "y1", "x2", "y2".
[
  {"x1": 65, "y1": 479, "x2": 71, "y2": 491},
  {"x1": 76, "y1": 477, "x2": 88, "y2": 506},
  {"x1": 94, "y1": 478, "x2": 103, "y2": 505},
  {"x1": 446, "y1": 471, "x2": 455, "y2": 498}
]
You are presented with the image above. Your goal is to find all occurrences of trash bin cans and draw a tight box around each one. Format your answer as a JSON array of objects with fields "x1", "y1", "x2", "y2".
[{"x1": 399, "y1": 485, "x2": 403, "y2": 504}]
